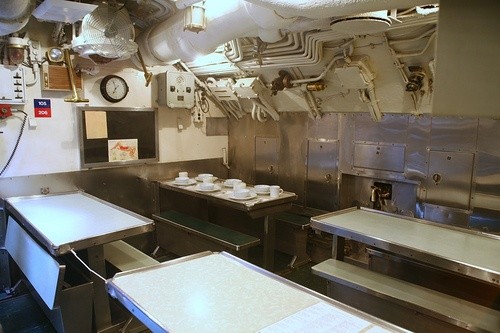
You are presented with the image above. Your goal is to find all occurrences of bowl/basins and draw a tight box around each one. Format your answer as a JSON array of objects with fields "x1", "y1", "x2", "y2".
[
  {"x1": 255, "y1": 185, "x2": 269, "y2": 192},
  {"x1": 227, "y1": 179, "x2": 238, "y2": 184},
  {"x1": 235, "y1": 189, "x2": 250, "y2": 197},
  {"x1": 201, "y1": 183, "x2": 214, "y2": 189},
  {"x1": 199, "y1": 174, "x2": 213, "y2": 179},
  {"x1": 175, "y1": 178, "x2": 189, "y2": 183}
]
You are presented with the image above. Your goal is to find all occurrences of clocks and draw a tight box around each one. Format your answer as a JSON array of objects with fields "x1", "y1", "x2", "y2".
[{"x1": 101, "y1": 74, "x2": 129, "y2": 103}]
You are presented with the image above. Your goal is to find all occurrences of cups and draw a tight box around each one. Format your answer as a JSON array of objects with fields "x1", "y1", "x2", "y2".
[
  {"x1": 233, "y1": 183, "x2": 246, "y2": 191},
  {"x1": 179, "y1": 172, "x2": 187, "y2": 178},
  {"x1": 270, "y1": 186, "x2": 283, "y2": 198},
  {"x1": 203, "y1": 177, "x2": 215, "y2": 183}
]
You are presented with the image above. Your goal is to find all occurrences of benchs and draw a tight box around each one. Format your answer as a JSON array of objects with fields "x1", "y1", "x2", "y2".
[
  {"x1": 152, "y1": 211, "x2": 262, "y2": 261},
  {"x1": 4, "y1": 216, "x2": 93, "y2": 333},
  {"x1": 311, "y1": 259, "x2": 500, "y2": 333},
  {"x1": 105, "y1": 241, "x2": 160, "y2": 271},
  {"x1": 277, "y1": 211, "x2": 312, "y2": 268}
]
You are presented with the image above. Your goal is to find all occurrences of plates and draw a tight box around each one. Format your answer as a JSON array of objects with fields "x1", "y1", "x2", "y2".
[
  {"x1": 230, "y1": 194, "x2": 257, "y2": 200},
  {"x1": 196, "y1": 177, "x2": 217, "y2": 181},
  {"x1": 224, "y1": 185, "x2": 232, "y2": 187},
  {"x1": 197, "y1": 188, "x2": 221, "y2": 192},
  {"x1": 173, "y1": 181, "x2": 197, "y2": 185},
  {"x1": 257, "y1": 192, "x2": 270, "y2": 195}
]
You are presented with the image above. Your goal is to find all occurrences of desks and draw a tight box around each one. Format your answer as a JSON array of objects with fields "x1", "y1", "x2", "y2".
[
  {"x1": 4, "y1": 189, "x2": 155, "y2": 324},
  {"x1": 105, "y1": 251, "x2": 415, "y2": 333},
  {"x1": 310, "y1": 206, "x2": 500, "y2": 286},
  {"x1": 156, "y1": 178, "x2": 298, "y2": 269}
]
